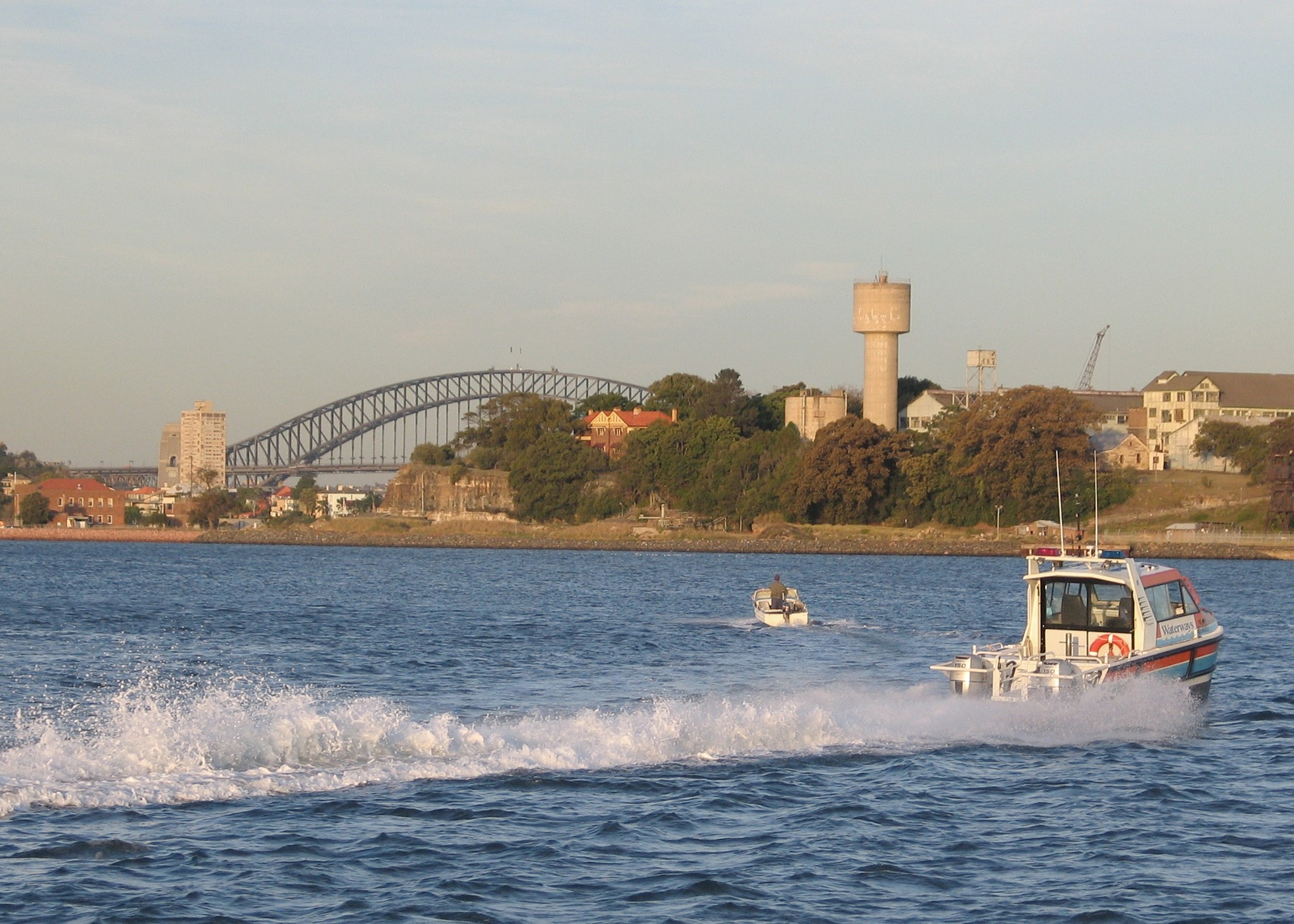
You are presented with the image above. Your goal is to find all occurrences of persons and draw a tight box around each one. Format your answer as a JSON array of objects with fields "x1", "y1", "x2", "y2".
[{"x1": 768, "y1": 574, "x2": 788, "y2": 609}]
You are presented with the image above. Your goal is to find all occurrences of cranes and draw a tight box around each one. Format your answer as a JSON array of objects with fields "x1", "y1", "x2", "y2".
[{"x1": 1073, "y1": 324, "x2": 1110, "y2": 390}]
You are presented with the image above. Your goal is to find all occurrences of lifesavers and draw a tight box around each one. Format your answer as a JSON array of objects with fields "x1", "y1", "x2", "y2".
[{"x1": 1088, "y1": 634, "x2": 1130, "y2": 660}]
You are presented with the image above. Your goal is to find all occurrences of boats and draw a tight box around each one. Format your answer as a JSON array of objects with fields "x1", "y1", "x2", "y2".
[
  {"x1": 751, "y1": 586, "x2": 808, "y2": 628},
  {"x1": 929, "y1": 449, "x2": 1224, "y2": 710}
]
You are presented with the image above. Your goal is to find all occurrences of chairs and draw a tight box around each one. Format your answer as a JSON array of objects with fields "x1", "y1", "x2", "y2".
[
  {"x1": 1117, "y1": 597, "x2": 1135, "y2": 630},
  {"x1": 1059, "y1": 594, "x2": 1089, "y2": 627}
]
